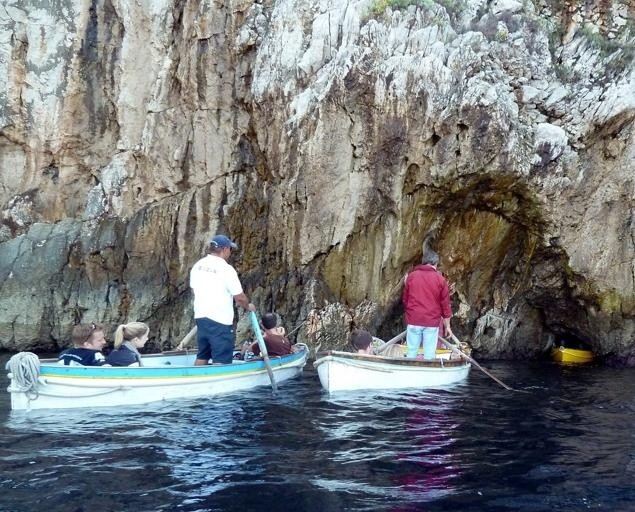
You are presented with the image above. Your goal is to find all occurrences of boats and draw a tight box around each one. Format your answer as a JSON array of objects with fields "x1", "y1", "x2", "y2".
[
  {"x1": 312, "y1": 341, "x2": 473, "y2": 395},
  {"x1": 5, "y1": 342, "x2": 309, "y2": 414},
  {"x1": 549, "y1": 345, "x2": 595, "y2": 365}
]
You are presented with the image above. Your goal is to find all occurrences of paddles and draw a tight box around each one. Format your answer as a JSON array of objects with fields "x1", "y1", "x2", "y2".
[
  {"x1": 438, "y1": 335, "x2": 512, "y2": 391},
  {"x1": 249, "y1": 311, "x2": 278, "y2": 392}
]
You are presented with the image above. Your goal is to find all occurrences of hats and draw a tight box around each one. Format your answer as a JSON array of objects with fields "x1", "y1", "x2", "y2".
[{"x1": 209, "y1": 235, "x2": 236, "y2": 248}]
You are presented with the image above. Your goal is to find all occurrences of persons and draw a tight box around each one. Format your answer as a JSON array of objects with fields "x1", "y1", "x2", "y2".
[
  {"x1": 101, "y1": 320, "x2": 151, "y2": 368},
  {"x1": 168, "y1": 396, "x2": 251, "y2": 510},
  {"x1": 421, "y1": 271, "x2": 451, "y2": 348},
  {"x1": 240, "y1": 311, "x2": 292, "y2": 363},
  {"x1": 57, "y1": 321, "x2": 107, "y2": 367},
  {"x1": 187, "y1": 234, "x2": 258, "y2": 365},
  {"x1": 348, "y1": 327, "x2": 377, "y2": 363},
  {"x1": 389, "y1": 392, "x2": 460, "y2": 511},
  {"x1": 401, "y1": 248, "x2": 455, "y2": 362},
  {"x1": 335, "y1": 329, "x2": 363, "y2": 360}
]
